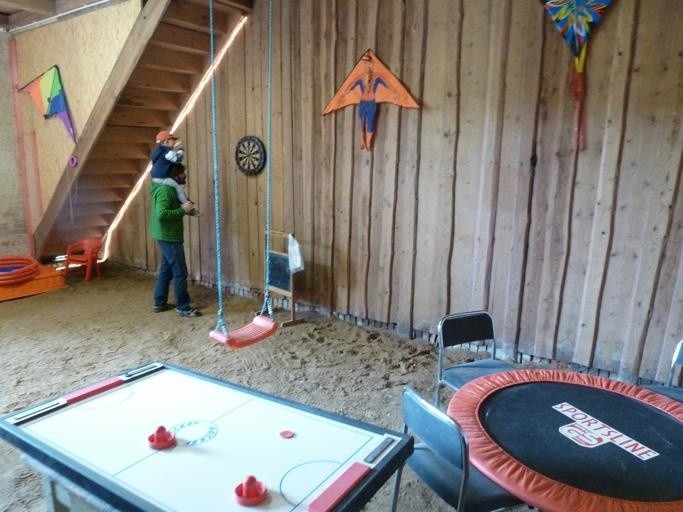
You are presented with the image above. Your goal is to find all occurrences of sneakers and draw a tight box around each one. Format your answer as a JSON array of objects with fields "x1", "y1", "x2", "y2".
[
  {"x1": 151, "y1": 303, "x2": 174, "y2": 312},
  {"x1": 188, "y1": 208, "x2": 199, "y2": 215},
  {"x1": 175, "y1": 309, "x2": 201, "y2": 317}
]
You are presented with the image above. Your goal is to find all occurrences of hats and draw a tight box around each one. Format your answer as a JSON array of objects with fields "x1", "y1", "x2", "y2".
[{"x1": 155, "y1": 130, "x2": 177, "y2": 143}]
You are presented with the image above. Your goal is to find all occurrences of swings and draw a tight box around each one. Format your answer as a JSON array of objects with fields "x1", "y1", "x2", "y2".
[{"x1": 209, "y1": 1, "x2": 278, "y2": 349}]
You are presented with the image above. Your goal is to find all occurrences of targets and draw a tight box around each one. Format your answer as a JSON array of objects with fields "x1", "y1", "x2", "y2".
[{"x1": 235, "y1": 136, "x2": 265, "y2": 174}]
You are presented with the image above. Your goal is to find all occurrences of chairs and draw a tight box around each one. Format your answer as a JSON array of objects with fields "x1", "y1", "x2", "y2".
[
  {"x1": 389, "y1": 385, "x2": 534, "y2": 512},
  {"x1": 435, "y1": 310, "x2": 520, "y2": 410},
  {"x1": 640, "y1": 340, "x2": 683, "y2": 403},
  {"x1": 64, "y1": 238, "x2": 102, "y2": 282}
]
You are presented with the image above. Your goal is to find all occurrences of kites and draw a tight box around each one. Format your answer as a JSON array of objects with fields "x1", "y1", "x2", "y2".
[
  {"x1": 13, "y1": 63, "x2": 80, "y2": 148},
  {"x1": 321, "y1": 48, "x2": 421, "y2": 154},
  {"x1": 539, "y1": 0, "x2": 617, "y2": 153}
]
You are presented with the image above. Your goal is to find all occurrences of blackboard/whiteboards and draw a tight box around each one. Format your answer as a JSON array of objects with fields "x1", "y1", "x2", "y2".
[{"x1": 264, "y1": 250, "x2": 295, "y2": 297}]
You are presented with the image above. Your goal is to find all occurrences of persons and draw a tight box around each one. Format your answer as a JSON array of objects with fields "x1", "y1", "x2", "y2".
[
  {"x1": 147, "y1": 163, "x2": 202, "y2": 318},
  {"x1": 151, "y1": 130, "x2": 199, "y2": 216}
]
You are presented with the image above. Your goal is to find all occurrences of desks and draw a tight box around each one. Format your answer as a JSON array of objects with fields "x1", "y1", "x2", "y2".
[
  {"x1": 0, "y1": 360, "x2": 415, "y2": 512},
  {"x1": 447, "y1": 369, "x2": 683, "y2": 512}
]
